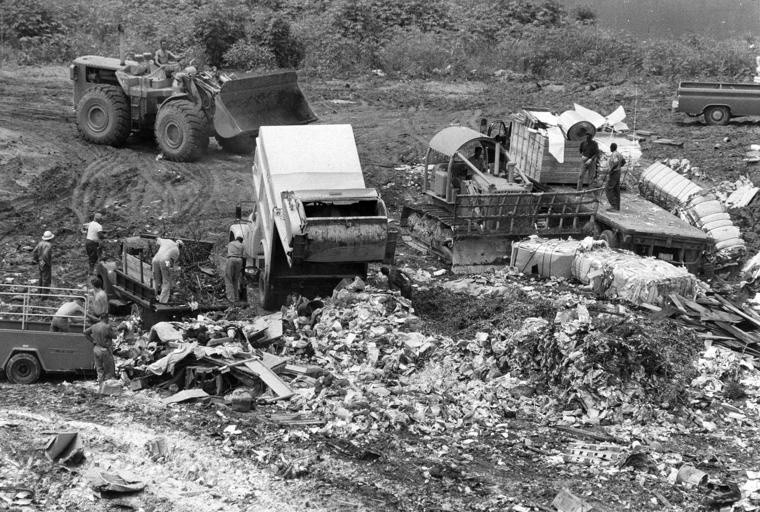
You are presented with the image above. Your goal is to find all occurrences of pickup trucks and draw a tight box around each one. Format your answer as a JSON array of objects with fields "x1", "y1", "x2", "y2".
[{"x1": 673, "y1": 76, "x2": 760, "y2": 128}]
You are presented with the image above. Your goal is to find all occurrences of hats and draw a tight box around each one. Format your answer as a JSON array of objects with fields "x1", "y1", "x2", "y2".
[{"x1": 42, "y1": 231, "x2": 55, "y2": 240}]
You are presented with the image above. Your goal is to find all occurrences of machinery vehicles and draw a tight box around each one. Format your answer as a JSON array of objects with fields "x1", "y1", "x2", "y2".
[
  {"x1": 403, "y1": 124, "x2": 606, "y2": 277},
  {"x1": 66, "y1": 21, "x2": 320, "y2": 163}
]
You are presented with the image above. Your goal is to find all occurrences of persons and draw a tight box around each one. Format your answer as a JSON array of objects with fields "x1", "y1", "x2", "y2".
[
  {"x1": 604, "y1": 143, "x2": 626, "y2": 212},
  {"x1": 224, "y1": 236, "x2": 244, "y2": 304},
  {"x1": 33, "y1": 230, "x2": 55, "y2": 301},
  {"x1": 151, "y1": 236, "x2": 185, "y2": 304},
  {"x1": 84, "y1": 213, "x2": 104, "y2": 276},
  {"x1": 465, "y1": 146, "x2": 486, "y2": 180},
  {"x1": 83, "y1": 313, "x2": 118, "y2": 388},
  {"x1": 154, "y1": 39, "x2": 181, "y2": 79},
  {"x1": 48, "y1": 296, "x2": 100, "y2": 332},
  {"x1": 576, "y1": 133, "x2": 599, "y2": 191},
  {"x1": 89, "y1": 278, "x2": 108, "y2": 317}
]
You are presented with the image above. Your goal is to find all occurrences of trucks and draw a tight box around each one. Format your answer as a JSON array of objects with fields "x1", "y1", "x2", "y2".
[
  {"x1": 488, "y1": 113, "x2": 719, "y2": 277},
  {"x1": 95, "y1": 228, "x2": 233, "y2": 336},
  {"x1": 229, "y1": 123, "x2": 414, "y2": 308},
  {"x1": 0, "y1": 282, "x2": 110, "y2": 387}
]
